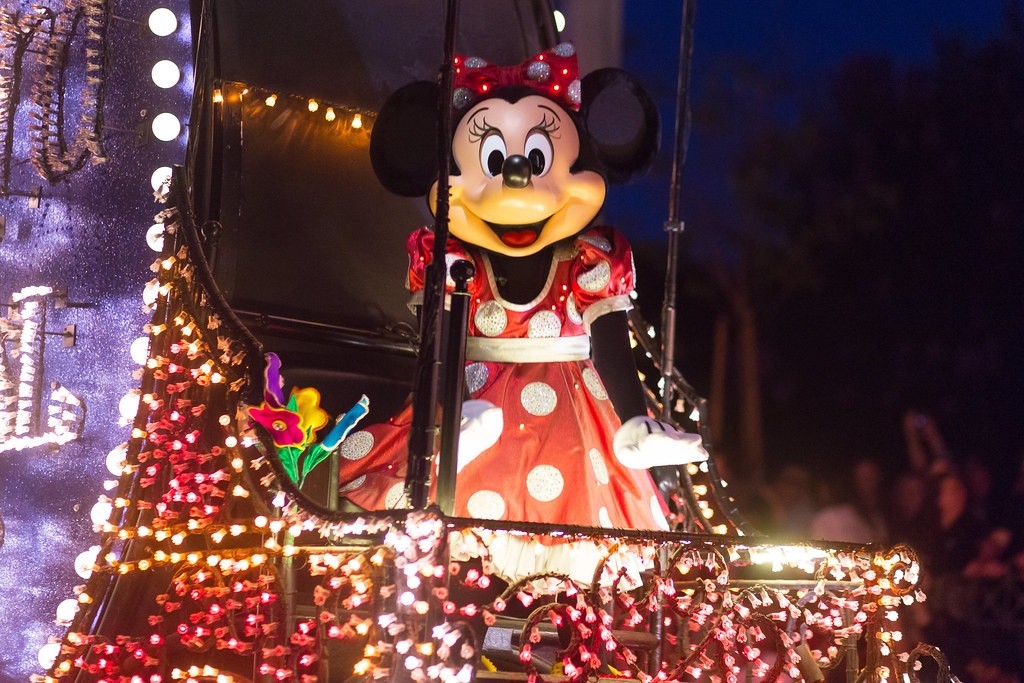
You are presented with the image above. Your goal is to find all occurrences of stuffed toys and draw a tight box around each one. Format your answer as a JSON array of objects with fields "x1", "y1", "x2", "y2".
[{"x1": 336, "y1": 41, "x2": 709, "y2": 532}]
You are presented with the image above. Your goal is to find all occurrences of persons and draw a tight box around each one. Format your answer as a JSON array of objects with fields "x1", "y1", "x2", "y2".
[{"x1": 714, "y1": 408, "x2": 1024, "y2": 683}]
